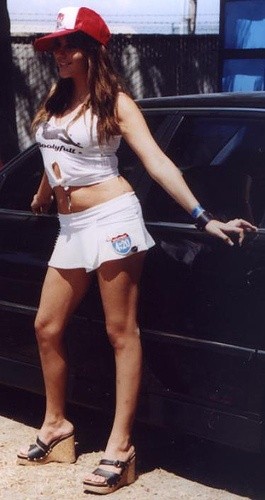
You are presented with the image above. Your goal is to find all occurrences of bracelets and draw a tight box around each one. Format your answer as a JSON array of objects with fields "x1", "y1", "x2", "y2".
[
  {"x1": 196, "y1": 210, "x2": 215, "y2": 225},
  {"x1": 191, "y1": 205, "x2": 205, "y2": 218}
]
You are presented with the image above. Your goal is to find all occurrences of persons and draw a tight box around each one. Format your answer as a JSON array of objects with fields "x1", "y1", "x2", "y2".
[{"x1": 14, "y1": 6, "x2": 260, "y2": 493}]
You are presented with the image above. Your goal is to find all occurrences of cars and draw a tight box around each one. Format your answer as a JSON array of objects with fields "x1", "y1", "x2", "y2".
[{"x1": 0, "y1": 92, "x2": 265, "y2": 456}]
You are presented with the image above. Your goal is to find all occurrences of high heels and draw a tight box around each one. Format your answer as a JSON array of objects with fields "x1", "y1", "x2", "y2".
[
  {"x1": 84, "y1": 449, "x2": 136, "y2": 496},
  {"x1": 16, "y1": 435, "x2": 76, "y2": 466}
]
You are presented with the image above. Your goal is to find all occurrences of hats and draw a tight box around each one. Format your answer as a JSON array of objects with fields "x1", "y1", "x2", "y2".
[{"x1": 34, "y1": 7, "x2": 111, "y2": 52}]
